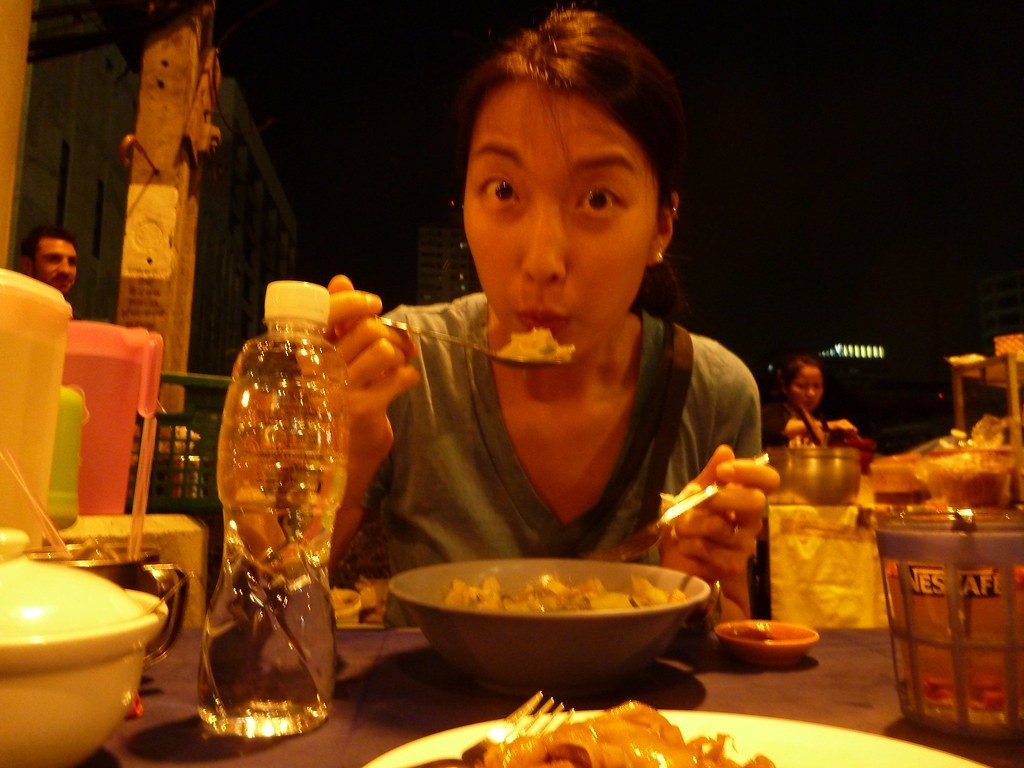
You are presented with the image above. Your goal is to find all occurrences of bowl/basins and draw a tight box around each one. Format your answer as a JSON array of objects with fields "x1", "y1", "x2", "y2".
[
  {"x1": 783, "y1": 447, "x2": 860, "y2": 505},
  {"x1": 387, "y1": 558, "x2": 710, "y2": 701},
  {"x1": 0, "y1": 527, "x2": 168, "y2": 768}
]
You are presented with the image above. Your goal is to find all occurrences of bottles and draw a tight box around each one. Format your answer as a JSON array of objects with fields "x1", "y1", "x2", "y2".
[{"x1": 193, "y1": 280, "x2": 350, "y2": 738}]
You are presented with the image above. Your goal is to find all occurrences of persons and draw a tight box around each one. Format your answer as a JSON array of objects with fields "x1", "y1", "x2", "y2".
[
  {"x1": 295, "y1": 10, "x2": 781, "y2": 617},
  {"x1": 763, "y1": 358, "x2": 858, "y2": 446},
  {"x1": 21, "y1": 228, "x2": 77, "y2": 293}
]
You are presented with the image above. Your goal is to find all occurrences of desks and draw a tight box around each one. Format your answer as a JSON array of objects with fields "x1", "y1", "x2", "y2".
[{"x1": 74, "y1": 628, "x2": 1024, "y2": 768}]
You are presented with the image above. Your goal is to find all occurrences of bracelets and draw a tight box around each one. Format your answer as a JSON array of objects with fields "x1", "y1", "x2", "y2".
[{"x1": 821, "y1": 420, "x2": 830, "y2": 431}]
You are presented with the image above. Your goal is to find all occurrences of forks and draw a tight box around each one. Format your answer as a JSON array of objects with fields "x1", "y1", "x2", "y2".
[
  {"x1": 465, "y1": 691, "x2": 577, "y2": 742},
  {"x1": 582, "y1": 453, "x2": 771, "y2": 560}
]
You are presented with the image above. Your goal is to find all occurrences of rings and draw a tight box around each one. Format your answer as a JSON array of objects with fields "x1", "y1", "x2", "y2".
[{"x1": 730, "y1": 523, "x2": 738, "y2": 549}]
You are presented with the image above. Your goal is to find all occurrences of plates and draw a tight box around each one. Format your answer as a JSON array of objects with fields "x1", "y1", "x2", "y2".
[
  {"x1": 716, "y1": 618, "x2": 819, "y2": 668},
  {"x1": 359, "y1": 710, "x2": 997, "y2": 768}
]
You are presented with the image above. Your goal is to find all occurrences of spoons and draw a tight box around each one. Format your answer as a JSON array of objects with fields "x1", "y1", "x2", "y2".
[{"x1": 376, "y1": 314, "x2": 574, "y2": 368}]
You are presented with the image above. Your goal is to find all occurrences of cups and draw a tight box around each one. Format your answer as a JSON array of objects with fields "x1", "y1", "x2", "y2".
[
  {"x1": 24, "y1": 547, "x2": 190, "y2": 670},
  {"x1": 870, "y1": 463, "x2": 931, "y2": 512},
  {"x1": 896, "y1": 448, "x2": 1012, "y2": 509}
]
